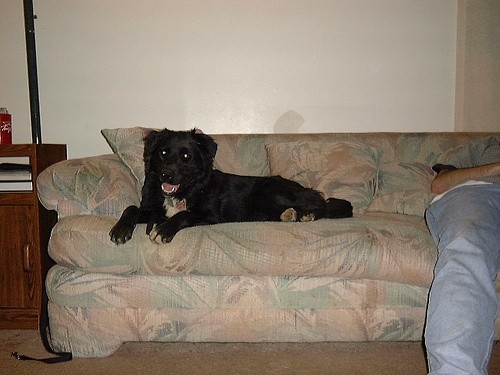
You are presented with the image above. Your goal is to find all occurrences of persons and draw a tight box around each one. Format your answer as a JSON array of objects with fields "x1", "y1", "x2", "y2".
[{"x1": 423, "y1": 135, "x2": 500, "y2": 375}]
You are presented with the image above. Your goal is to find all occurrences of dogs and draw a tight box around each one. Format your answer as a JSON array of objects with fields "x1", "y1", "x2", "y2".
[{"x1": 109, "y1": 128, "x2": 353, "y2": 246}]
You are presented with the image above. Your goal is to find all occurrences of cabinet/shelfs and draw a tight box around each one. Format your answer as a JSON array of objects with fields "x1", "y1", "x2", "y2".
[{"x1": 1, "y1": 143, "x2": 67, "y2": 331}]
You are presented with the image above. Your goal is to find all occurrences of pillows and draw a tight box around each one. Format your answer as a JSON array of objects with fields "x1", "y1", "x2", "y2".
[
  {"x1": 263, "y1": 143, "x2": 380, "y2": 218},
  {"x1": 365, "y1": 160, "x2": 432, "y2": 216},
  {"x1": 101, "y1": 127, "x2": 169, "y2": 201}
]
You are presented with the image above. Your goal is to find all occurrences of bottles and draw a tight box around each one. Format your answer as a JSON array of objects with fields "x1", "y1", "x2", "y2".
[{"x1": 0, "y1": 108, "x2": 12, "y2": 145}]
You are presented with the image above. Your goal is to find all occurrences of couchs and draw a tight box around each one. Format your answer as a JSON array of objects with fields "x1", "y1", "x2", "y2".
[{"x1": 34, "y1": 127, "x2": 499, "y2": 358}]
